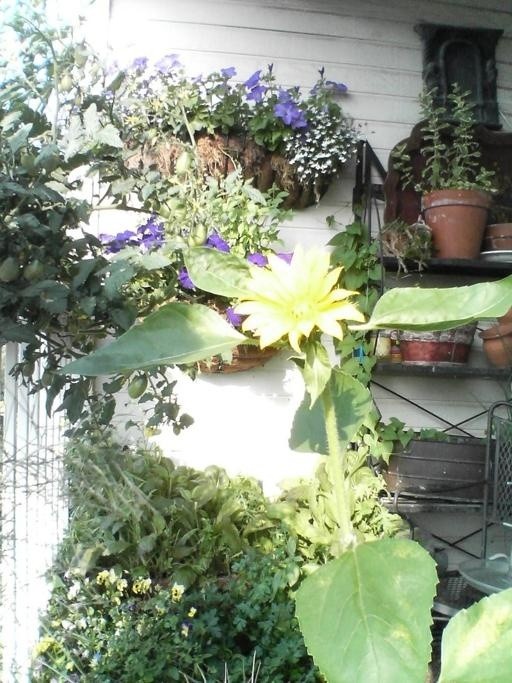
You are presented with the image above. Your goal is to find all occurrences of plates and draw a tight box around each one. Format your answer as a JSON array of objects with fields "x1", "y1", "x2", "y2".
[{"x1": 480, "y1": 250, "x2": 512, "y2": 263}]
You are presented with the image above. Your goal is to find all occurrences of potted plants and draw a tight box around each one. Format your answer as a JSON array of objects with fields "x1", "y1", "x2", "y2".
[
  {"x1": 391, "y1": 82, "x2": 500, "y2": 261},
  {"x1": 485, "y1": 205, "x2": 512, "y2": 250}
]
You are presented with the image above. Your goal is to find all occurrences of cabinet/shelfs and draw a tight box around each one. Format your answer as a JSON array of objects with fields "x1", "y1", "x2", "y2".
[{"x1": 353, "y1": 121, "x2": 512, "y2": 642}]
